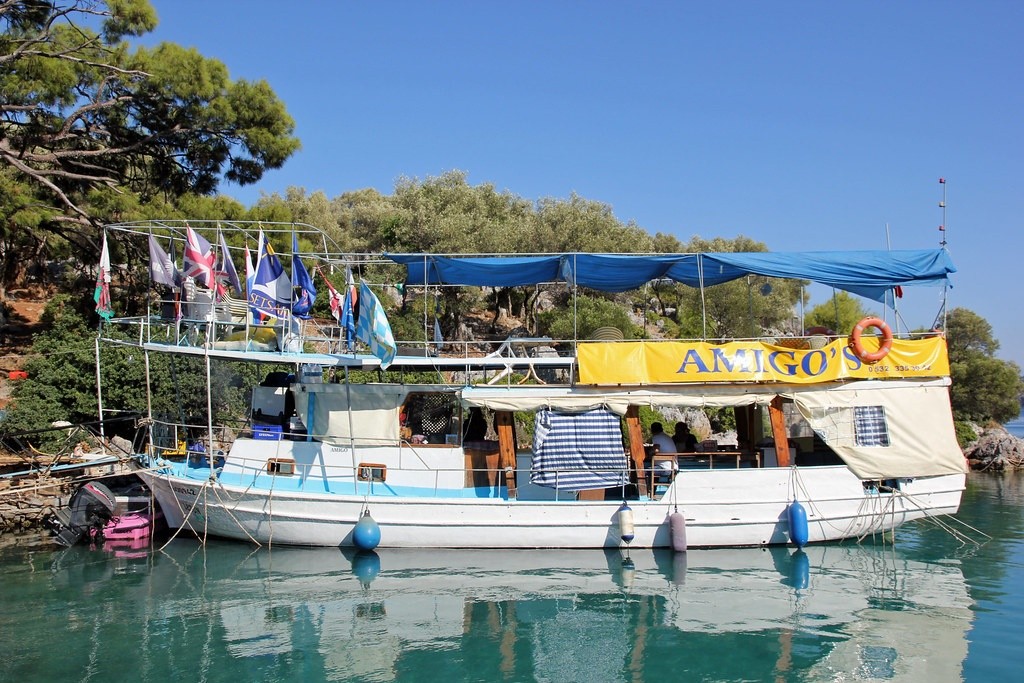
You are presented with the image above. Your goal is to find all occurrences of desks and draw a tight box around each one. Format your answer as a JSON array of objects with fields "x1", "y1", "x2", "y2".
[{"x1": 511, "y1": 341, "x2": 554, "y2": 385}]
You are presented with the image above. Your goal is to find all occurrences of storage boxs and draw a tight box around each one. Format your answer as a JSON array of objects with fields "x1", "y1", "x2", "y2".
[
  {"x1": 290, "y1": 417, "x2": 308, "y2": 441},
  {"x1": 760, "y1": 447, "x2": 798, "y2": 468},
  {"x1": 253, "y1": 387, "x2": 294, "y2": 424},
  {"x1": 252, "y1": 425, "x2": 284, "y2": 440}
]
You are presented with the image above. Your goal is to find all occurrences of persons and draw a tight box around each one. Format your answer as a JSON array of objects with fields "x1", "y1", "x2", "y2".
[
  {"x1": 642, "y1": 422, "x2": 679, "y2": 476},
  {"x1": 671, "y1": 422, "x2": 698, "y2": 453}
]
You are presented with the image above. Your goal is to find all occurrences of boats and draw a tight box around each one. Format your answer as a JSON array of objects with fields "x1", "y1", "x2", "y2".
[{"x1": 94, "y1": 218, "x2": 970, "y2": 550}]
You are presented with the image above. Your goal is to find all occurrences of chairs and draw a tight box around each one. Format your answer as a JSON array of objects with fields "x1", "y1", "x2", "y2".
[
  {"x1": 478, "y1": 335, "x2": 518, "y2": 386},
  {"x1": 651, "y1": 456, "x2": 675, "y2": 500},
  {"x1": 183, "y1": 282, "x2": 252, "y2": 349}
]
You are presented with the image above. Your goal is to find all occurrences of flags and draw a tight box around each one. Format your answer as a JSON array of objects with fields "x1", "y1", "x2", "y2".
[
  {"x1": 94, "y1": 227, "x2": 115, "y2": 325},
  {"x1": 341, "y1": 279, "x2": 397, "y2": 371},
  {"x1": 148, "y1": 226, "x2": 317, "y2": 320}
]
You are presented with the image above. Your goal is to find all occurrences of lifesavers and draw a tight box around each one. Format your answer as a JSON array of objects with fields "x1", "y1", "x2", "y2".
[{"x1": 848, "y1": 316, "x2": 893, "y2": 365}]
